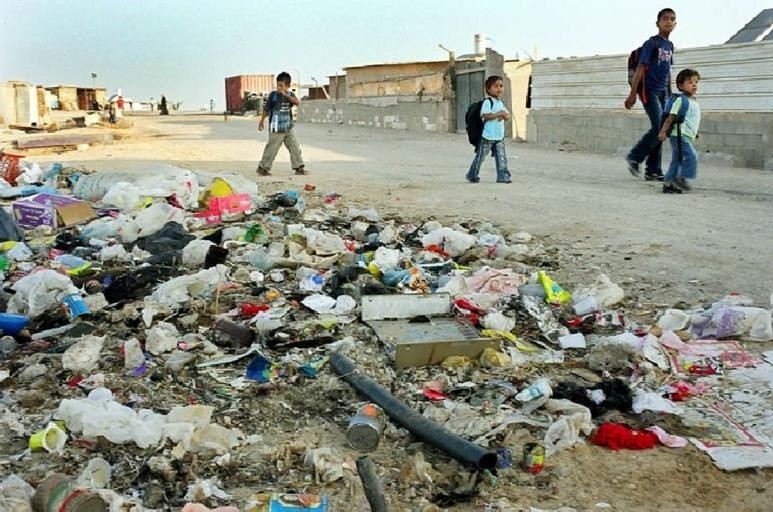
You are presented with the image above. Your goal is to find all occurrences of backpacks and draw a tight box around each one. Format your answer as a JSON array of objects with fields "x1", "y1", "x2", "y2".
[
  {"x1": 661, "y1": 93, "x2": 689, "y2": 136},
  {"x1": 465, "y1": 97, "x2": 493, "y2": 145},
  {"x1": 627, "y1": 38, "x2": 656, "y2": 94}
]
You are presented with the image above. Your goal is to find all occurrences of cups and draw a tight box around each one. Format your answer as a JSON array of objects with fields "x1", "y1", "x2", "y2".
[{"x1": 28, "y1": 428, "x2": 67, "y2": 454}]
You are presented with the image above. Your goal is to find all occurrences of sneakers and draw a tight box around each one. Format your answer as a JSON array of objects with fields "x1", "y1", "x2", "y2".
[
  {"x1": 257, "y1": 167, "x2": 272, "y2": 175},
  {"x1": 644, "y1": 171, "x2": 664, "y2": 181},
  {"x1": 625, "y1": 153, "x2": 640, "y2": 176},
  {"x1": 663, "y1": 176, "x2": 691, "y2": 194},
  {"x1": 295, "y1": 168, "x2": 308, "y2": 175}
]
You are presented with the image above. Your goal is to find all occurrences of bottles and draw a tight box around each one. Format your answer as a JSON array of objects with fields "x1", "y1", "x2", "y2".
[{"x1": 298, "y1": 274, "x2": 323, "y2": 293}]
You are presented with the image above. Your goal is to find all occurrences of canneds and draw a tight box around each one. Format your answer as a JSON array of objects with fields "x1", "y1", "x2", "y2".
[
  {"x1": 522, "y1": 442, "x2": 545, "y2": 474},
  {"x1": 346, "y1": 404, "x2": 384, "y2": 451},
  {"x1": 61, "y1": 294, "x2": 91, "y2": 322},
  {"x1": 34, "y1": 472, "x2": 105, "y2": 512}
]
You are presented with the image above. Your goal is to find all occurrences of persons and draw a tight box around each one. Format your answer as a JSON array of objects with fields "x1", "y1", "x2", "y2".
[
  {"x1": 117, "y1": 95, "x2": 124, "y2": 114},
  {"x1": 256, "y1": 71, "x2": 311, "y2": 175},
  {"x1": 160, "y1": 93, "x2": 169, "y2": 115},
  {"x1": 465, "y1": 74, "x2": 512, "y2": 184},
  {"x1": 108, "y1": 102, "x2": 116, "y2": 123},
  {"x1": 623, "y1": 7, "x2": 678, "y2": 182},
  {"x1": 658, "y1": 68, "x2": 703, "y2": 195}
]
